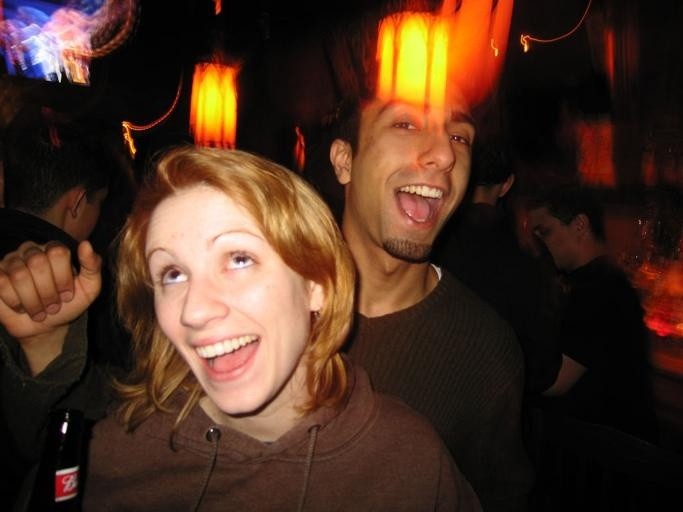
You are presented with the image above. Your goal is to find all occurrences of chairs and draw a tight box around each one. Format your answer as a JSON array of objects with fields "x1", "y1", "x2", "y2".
[{"x1": 526, "y1": 407, "x2": 682, "y2": 512}]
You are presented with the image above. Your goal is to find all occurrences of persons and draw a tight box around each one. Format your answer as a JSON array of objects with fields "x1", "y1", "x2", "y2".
[
  {"x1": 0, "y1": 63, "x2": 537, "y2": 512},
  {"x1": 74, "y1": 140, "x2": 487, "y2": 511},
  {"x1": 438, "y1": 115, "x2": 560, "y2": 394},
  {"x1": 525, "y1": 184, "x2": 663, "y2": 446},
  {"x1": 0, "y1": 97, "x2": 118, "y2": 512}
]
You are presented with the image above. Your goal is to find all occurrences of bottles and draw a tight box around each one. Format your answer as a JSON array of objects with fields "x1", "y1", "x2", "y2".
[{"x1": 40, "y1": 408, "x2": 85, "y2": 511}]
